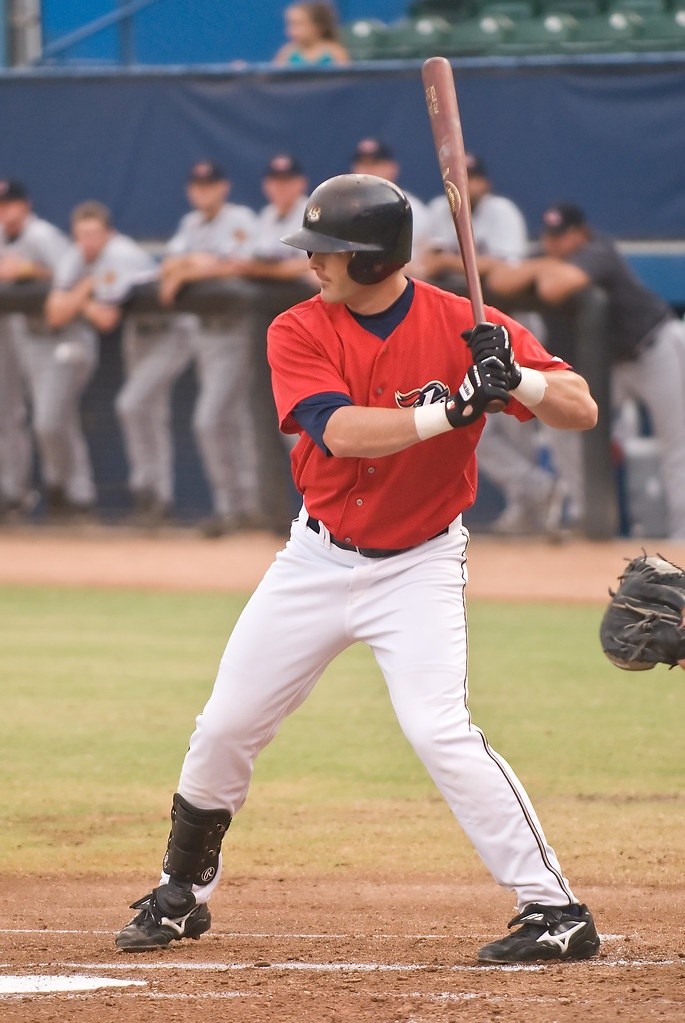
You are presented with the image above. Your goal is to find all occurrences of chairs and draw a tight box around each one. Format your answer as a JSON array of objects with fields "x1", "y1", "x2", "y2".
[{"x1": 335, "y1": 0, "x2": 685, "y2": 60}]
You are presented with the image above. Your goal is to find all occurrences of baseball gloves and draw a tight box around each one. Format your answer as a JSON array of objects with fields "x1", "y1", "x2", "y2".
[{"x1": 599, "y1": 546, "x2": 685, "y2": 672}]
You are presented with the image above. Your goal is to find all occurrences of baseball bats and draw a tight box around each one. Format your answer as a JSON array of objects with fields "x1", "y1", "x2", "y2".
[{"x1": 419, "y1": 54, "x2": 506, "y2": 415}]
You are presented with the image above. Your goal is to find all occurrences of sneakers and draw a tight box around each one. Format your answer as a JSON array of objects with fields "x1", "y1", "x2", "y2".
[
  {"x1": 114, "y1": 887, "x2": 211, "y2": 952},
  {"x1": 477, "y1": 904, "x2": 600, "y2": 963}
]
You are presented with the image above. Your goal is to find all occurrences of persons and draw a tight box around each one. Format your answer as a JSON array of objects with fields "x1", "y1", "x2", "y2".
[
  {"x1": 159, "y1": 163, "x2": 267, "y2": 535},
  {"x1": 425, "y1": 157, "x2": 583, "y2": 534},
  {"x1": 487, "y1": 203, "x2": 685, "y2": 538},
  {"x1": 233, "y1": 154, "x2": 321, "y2": 281},
  {"x1": 356, "y1": 138, "x2": 433, "y2": 278},
  {"x1": 275, "y1": 0, "x2": 347, "y2": 64},
  {"x1": 48, "y1": 202, "x2": 196, "y2": 524},
  {"x1": 116, "y1": 173, "x2": 601, "y2": 962},
  {"x1": 0, "y1": 178, "x2": 97, "y2": 514}
]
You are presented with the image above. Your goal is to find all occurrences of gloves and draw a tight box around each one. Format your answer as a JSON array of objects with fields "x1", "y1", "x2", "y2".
[
  {"x1": 461, "y1": 322, "x2": 522, "y2": 390},
  {"x1": 444, "y1": 354, "x2": 507, "y2": 428}
]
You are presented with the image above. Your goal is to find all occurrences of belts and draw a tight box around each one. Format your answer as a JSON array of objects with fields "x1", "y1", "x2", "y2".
[{"x1": 306, "y1": 515, "x2": 449, "y2": 558}]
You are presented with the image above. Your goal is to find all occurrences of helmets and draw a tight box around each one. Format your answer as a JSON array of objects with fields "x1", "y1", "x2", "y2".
[{"x1": 280, "y1": 173, "x2": 413, "y2": 285}]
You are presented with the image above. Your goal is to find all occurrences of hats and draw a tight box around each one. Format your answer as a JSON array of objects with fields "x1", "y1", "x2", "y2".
[
  {"x1": 0, "y1": 182, "x2": 26, "y2": 198},
  {"x1": 542, "y1": 202, "x2": 584, "y2": 234},
  {"x1": 268, "y1": 156, "x2": 300, "y2": 179},
  {"x1": 190, "y1": 162, "x2": 224, "y2": 182},
  {"x1": 467, "y1": 153, "x2": 486, "y2": 173},
  {"x1": 351, "y1": 139, "x2": 395, "y2": 160}
]
